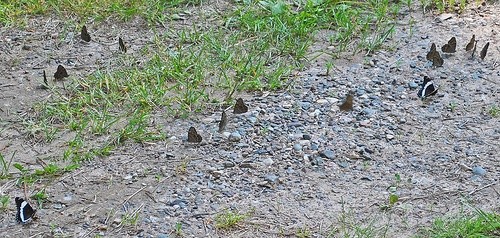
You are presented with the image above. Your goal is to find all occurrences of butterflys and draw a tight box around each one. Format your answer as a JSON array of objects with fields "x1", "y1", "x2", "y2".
[
  {"x1": 231, "y1": 98, "x2": 248, "y2": 115},
  {"x1": 80, "y1": 25, "x2": 92, "y2": 42},
  {"x1": 417, "y1": 75, "x2": 441, "y2": 99},
  {"x1": 52, "y1": 65, "x2": 70, "y2": 80},
  {"x1": 217, "y1": 110, "x2": 228, "y2": 133},
  {"x1": 119, "y1": 37, "x2": 128, "y2": 53},
  {"x1": 338, "y1": 89, "x2": 354, "y2": 111},
  {"x1": 15, "y1": 196, "x2": 39, "y2": 227},
  {"x1": 426, "y1": 34, "x2": 490, "y2": 69},
  {"x1": 185, "y1": 126, "x2": 203, "y2": 143}
]
